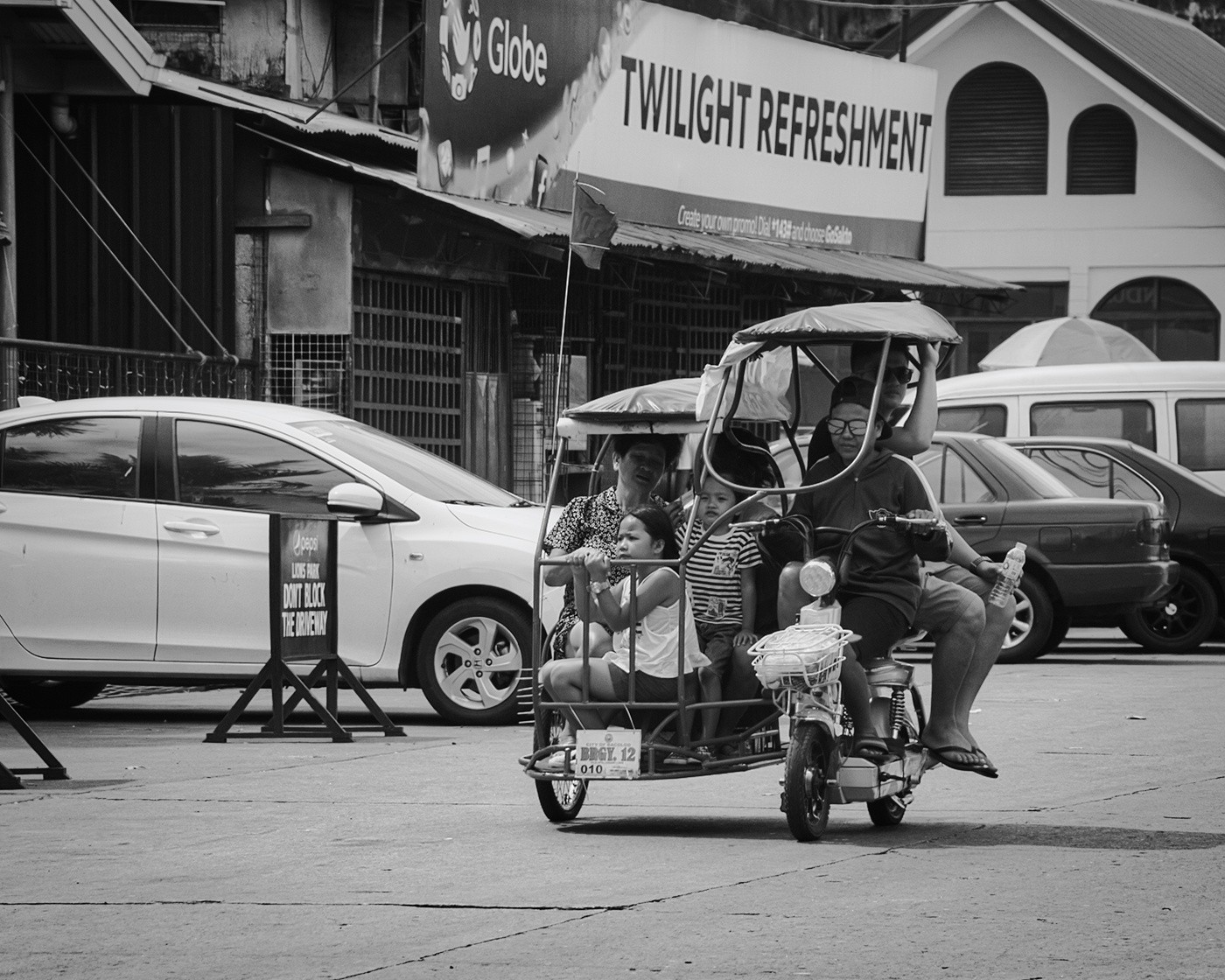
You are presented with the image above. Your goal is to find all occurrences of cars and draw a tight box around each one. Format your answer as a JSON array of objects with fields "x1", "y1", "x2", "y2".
[
  {"x1": 675, "y1": 434, "x2": 1225, "y2": 663},
  {"x1": 0, "y1": 396, "x2": 567, "y2": 725}
]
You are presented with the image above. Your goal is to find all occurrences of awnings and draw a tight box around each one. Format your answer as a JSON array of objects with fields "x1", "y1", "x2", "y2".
[
  {"x1": 153, "y1": 63, "x2": 420, "y2": 161},
  {"x1": 238, "y1": 120, "x2": 1028, "y2": 300}
]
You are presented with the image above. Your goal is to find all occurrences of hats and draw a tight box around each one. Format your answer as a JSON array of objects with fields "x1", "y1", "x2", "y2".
[{"x1": 829, "y1": 376, "x2": 893, "y2": 441}]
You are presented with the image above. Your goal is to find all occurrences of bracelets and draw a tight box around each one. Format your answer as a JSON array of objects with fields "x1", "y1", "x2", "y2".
[{"x1": 970, "y1": 556, "x2": 991, "y2": 572}]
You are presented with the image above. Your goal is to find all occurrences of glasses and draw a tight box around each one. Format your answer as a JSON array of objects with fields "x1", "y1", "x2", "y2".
[
  {"x1": 826, "y1": 418, "x2": 869, "y2": 436},
  {"x1": 855, "y1": 366, "x2": 914, "y2": 385}
]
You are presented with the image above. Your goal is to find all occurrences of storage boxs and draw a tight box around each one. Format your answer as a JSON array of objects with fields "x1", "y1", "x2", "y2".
[{"x1": 575, "y1": 727, "x2": 642, "y2": 779}]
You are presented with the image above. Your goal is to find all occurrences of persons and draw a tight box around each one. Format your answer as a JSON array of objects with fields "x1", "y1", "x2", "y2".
[
  {"x1": 806, "y1": 342, "x2": 1023, "y2": 778},
  {"x1": 762, "y1": 374, "x2": 953, "y2": 759},
  {"x1": 518, "y1": 427, "x2": 781, "y2": 773}
]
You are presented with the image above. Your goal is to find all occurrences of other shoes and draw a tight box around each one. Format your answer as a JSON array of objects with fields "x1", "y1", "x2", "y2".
[
  {"x1": 552, "y1": 734, "x2": 576, "y2": 756},
  {"x1": 718, "y1": 743, "x2": 740, "y2": 758},
  {"x1": 663, "y1": 744, "x2": 690, "y2": 764},
  {"x1": 687, "y1": 746, "x2": 717, "y2": 762},
  {"x1": 535, "y1": 748, "x2": 576, "y2": 771}
]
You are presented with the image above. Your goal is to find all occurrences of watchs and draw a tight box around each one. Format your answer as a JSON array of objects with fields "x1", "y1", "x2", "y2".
[{"x1": 588, "y1": 581, "x2": 610, "y2": 594}]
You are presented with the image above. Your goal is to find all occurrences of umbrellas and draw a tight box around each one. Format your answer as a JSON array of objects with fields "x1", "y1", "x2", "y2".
[{"x1": 978, "y1": 316, "x2": 1162, "y2": 371}]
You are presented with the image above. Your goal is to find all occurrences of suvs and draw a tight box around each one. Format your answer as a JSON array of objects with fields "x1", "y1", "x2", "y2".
[{"x1": 887, "y1": 361, "x2": 1225, "y2": 494}]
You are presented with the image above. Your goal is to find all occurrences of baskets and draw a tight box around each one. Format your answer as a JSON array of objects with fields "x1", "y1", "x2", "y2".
[{"x1": 746, "y1": 624, "x2": 853, "y2": 689}]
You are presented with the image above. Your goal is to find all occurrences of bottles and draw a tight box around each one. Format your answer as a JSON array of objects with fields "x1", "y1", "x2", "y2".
[{"x1": 988, "y1": 542, "x2": 1027, "y2": 608}]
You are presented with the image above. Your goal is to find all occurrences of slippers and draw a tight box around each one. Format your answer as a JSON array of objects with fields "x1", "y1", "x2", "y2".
[
  {"x1": 911, "y1": 743, "x2": 985, "y2": 770},
  {"x1": 971, "y1": 745, "x2": 999, "y2": 779},
  {"x1": 853, "y1": 735, "x2": 889, "y2": 762}
]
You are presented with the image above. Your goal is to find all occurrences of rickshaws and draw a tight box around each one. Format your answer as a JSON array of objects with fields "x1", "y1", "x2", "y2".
[{"x1": 518, "y1": 301, "x2": 965, "y2": 842}]
